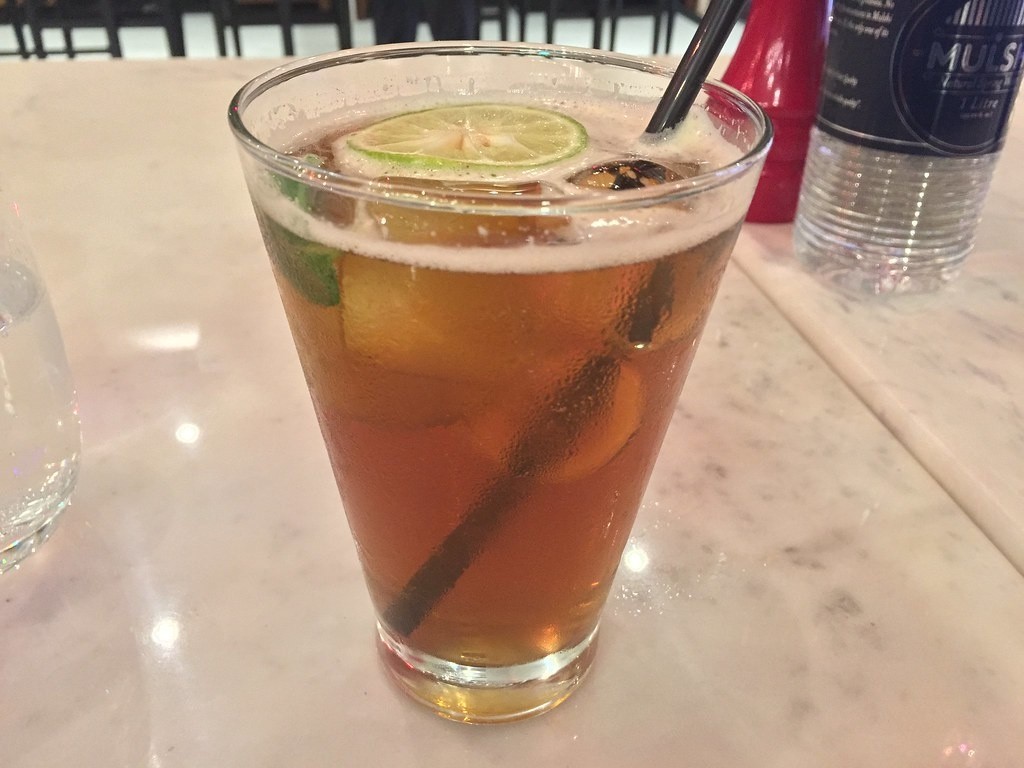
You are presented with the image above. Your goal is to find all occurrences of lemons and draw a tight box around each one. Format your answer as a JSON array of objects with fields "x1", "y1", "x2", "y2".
[{"x1": 345, "y1": 104, "x2": 591, "y2": 171}]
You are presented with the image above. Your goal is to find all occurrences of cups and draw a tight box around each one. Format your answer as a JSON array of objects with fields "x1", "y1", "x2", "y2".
[{"x1": 229, "y1": 39, "x2": 774, "y2": 725}]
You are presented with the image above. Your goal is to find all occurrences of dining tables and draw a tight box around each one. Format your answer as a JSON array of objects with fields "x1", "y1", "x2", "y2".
[
  {"x1": 591, "y1": 41, "x2": 1024, "y2": 571},
  {"x1": 1, "y1": 52, "x2": 1024, "y2": 768}
]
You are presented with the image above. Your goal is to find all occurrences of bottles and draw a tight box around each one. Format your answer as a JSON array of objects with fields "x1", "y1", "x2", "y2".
[{"x1": 791, "y1": 1, "x2": 1022, "y2": 305}]
